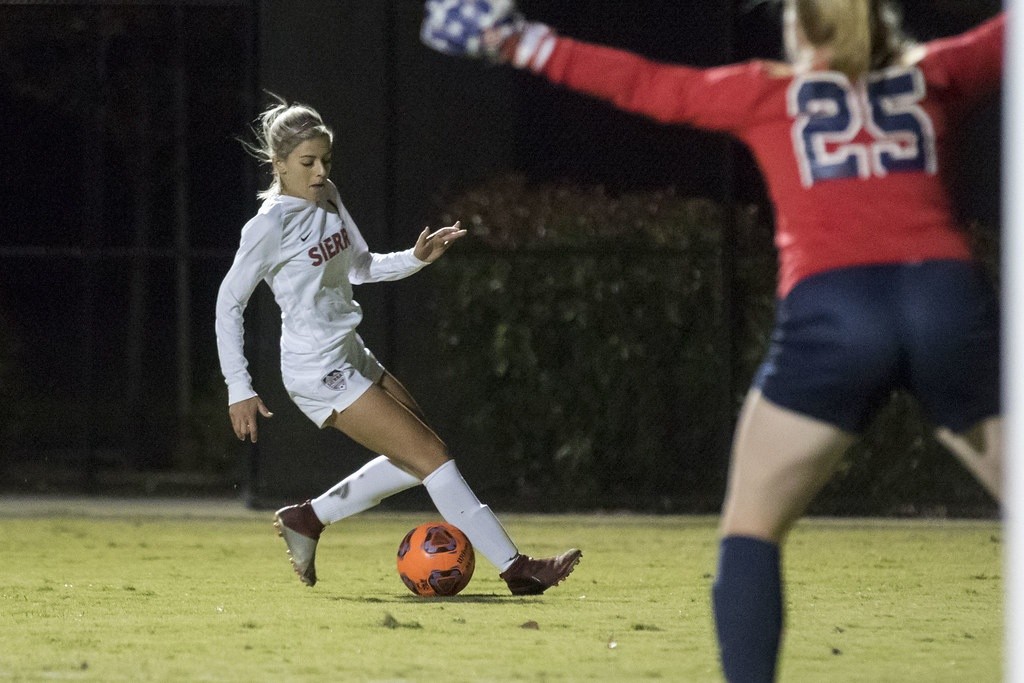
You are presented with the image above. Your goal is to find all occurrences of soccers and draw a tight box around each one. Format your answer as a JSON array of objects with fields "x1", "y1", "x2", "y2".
[{"x1": 393, "y1": 519, "x2": 478, "y2": 598}]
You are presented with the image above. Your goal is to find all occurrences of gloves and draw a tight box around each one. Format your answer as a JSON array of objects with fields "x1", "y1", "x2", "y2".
[{"x1": 419, "y1": 0, "x2": 526, "y2": 66}]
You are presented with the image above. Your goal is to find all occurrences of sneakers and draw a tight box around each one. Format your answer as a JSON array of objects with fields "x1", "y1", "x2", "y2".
[
  {"x1": 499, "y1": 550, "x2": 582, "y2": 594},
  {"x1": 273, "y1": 500, "x2": 324, "y2": 587}
]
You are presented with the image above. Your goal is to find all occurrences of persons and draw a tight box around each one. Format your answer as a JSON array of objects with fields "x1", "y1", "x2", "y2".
[
  {"x1": 419, "y1": 0, "x2": 1004, "y2": 683},
  {"x1": 214, "y1": 104, "x2": 583, "y2": 595}
]
